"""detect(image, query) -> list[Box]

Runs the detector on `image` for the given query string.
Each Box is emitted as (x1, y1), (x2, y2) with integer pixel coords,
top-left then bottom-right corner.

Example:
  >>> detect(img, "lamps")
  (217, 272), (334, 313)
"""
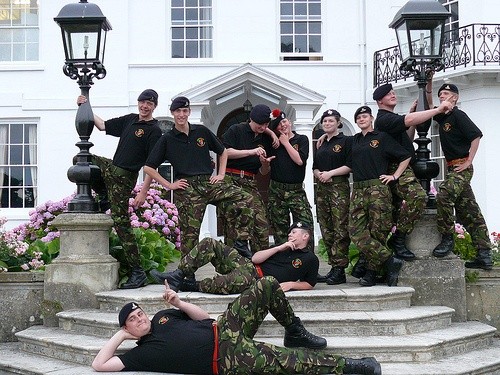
(244, 86), (253, 111)
(53, 0), (112, 213)
(388, 0), (451, 212)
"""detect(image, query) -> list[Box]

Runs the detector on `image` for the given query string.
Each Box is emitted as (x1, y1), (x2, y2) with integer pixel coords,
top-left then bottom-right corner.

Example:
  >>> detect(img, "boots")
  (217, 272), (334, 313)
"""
(387, 227), (416, 261)
(386, 254), (403, 286)
(351, 265), (364, 278)
(150, 266), (185, 292)
(344, 353), (381, 374)
(234, 238), (253, 258)
(432, 232), (454, 257)
(120, 264), (148, 287)
(317, 266), (346, 284)
(98, 187), (111, 212)
(464, 248), (493, 269)
(283, 317), (327, 349)
(359, 268), (377, 285)
(179, 278), (201, 291)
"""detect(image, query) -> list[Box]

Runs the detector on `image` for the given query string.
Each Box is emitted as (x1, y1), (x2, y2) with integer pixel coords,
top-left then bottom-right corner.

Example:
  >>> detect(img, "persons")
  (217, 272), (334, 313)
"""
(71, 88), (163, 289)
(353, 83), (452, 277)
(218, 104), (314, 252)
(312, 109), (353, 285)
(150, 222), (319, 297)
(316, 106), (412, 287)
(426, 79), (493, 271)
(144, 96), (255, 285)
(92, 275), (382, 375)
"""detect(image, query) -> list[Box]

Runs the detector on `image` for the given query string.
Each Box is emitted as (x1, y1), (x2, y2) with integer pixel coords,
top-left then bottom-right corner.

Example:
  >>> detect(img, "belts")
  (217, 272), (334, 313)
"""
(225, 172), (253, 179)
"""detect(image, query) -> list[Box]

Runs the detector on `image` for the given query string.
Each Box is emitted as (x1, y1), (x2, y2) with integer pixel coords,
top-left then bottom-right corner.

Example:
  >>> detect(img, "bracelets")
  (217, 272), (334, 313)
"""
(392, 175), (396, 180)
(426, 90), (432, 93)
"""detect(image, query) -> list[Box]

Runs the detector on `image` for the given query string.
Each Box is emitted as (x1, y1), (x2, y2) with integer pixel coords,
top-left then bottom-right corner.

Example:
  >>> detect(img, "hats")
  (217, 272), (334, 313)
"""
(373, 82), (391, 100)
(354, 105), (371, 122)
(170, 96), (190, 110)
(138, 88), (158, 101)
(269, 109), (286, 130)
(250, 103), (271, 124)
(119, 301), (139, 327)
(293, 220), (309, 227)
(437, 83), (458, 97)
(320, 109), (341, 123)
